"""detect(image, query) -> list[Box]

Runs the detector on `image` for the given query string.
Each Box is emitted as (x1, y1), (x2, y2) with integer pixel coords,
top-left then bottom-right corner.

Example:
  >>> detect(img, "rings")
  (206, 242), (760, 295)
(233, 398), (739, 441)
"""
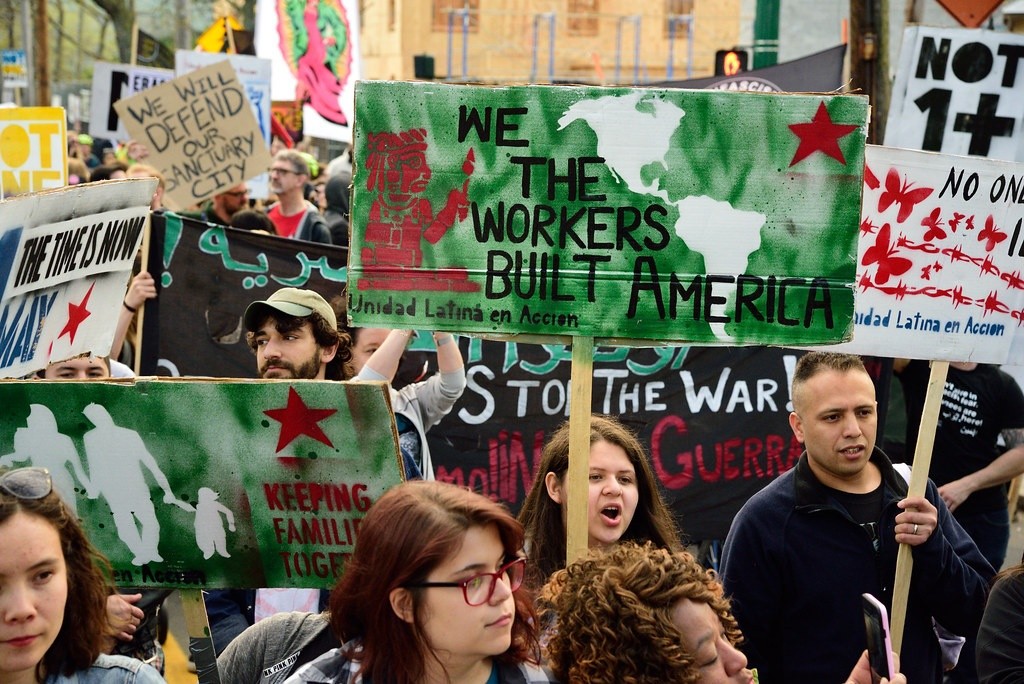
(913, 524), (918, 535)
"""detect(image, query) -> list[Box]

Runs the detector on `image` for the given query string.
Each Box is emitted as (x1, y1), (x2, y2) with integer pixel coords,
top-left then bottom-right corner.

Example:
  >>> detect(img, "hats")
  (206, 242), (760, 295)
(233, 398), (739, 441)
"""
(78, 134), (93, 145)
(244, 288), (337, 333)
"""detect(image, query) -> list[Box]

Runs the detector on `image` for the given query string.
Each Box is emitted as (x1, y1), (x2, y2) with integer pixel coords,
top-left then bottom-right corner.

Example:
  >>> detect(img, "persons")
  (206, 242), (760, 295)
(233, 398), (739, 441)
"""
(711, 349), (1002, 684)
(892, 358), (1024, 576)
(518, 414), (689, 635)
(976, 551), (1024, 684)
(1, 135), (547, 684)
(533, 539), (756, 684)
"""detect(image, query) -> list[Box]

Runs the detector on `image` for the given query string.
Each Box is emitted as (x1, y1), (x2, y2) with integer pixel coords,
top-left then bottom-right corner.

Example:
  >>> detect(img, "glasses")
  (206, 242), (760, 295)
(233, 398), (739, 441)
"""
(270, 168), (299, 177)
(0, 467), (52, 500)
(404, 557), (526, 606)
(226, 189), (247, 196)
